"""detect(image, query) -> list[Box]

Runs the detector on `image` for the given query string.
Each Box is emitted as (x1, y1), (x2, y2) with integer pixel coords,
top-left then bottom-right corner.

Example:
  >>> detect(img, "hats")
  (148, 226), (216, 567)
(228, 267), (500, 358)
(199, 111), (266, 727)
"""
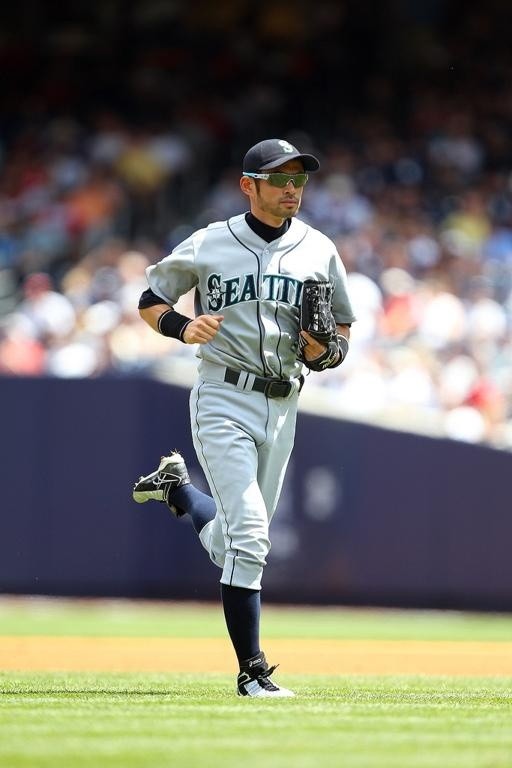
(244, 138), (321, 173)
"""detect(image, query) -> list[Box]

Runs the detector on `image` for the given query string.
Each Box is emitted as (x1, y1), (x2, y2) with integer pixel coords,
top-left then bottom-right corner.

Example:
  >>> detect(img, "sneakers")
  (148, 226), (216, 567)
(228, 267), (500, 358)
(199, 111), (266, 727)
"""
(236, 651), (293, 698)
(132, 454), (193, 519)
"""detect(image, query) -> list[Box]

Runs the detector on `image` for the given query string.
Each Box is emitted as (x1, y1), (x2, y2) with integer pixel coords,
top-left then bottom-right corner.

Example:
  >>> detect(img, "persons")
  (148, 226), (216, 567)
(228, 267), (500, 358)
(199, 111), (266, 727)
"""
(0, 1), (512, 452)
(131, 136), (356, 698)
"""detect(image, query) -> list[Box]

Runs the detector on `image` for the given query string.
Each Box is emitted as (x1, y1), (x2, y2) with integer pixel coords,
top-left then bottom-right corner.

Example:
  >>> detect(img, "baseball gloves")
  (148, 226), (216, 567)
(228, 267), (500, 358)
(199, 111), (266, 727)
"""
(298, 279), (338, 343)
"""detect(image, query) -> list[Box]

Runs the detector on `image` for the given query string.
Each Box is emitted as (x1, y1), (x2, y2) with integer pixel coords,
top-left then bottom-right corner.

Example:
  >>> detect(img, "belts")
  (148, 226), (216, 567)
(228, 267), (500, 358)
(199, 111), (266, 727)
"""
(224, 366), (304, 397)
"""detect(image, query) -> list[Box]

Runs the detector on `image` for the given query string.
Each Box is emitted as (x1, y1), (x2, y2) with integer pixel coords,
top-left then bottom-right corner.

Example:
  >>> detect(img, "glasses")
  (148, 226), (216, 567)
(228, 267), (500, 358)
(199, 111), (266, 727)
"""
(251, 173), (310, 188)
